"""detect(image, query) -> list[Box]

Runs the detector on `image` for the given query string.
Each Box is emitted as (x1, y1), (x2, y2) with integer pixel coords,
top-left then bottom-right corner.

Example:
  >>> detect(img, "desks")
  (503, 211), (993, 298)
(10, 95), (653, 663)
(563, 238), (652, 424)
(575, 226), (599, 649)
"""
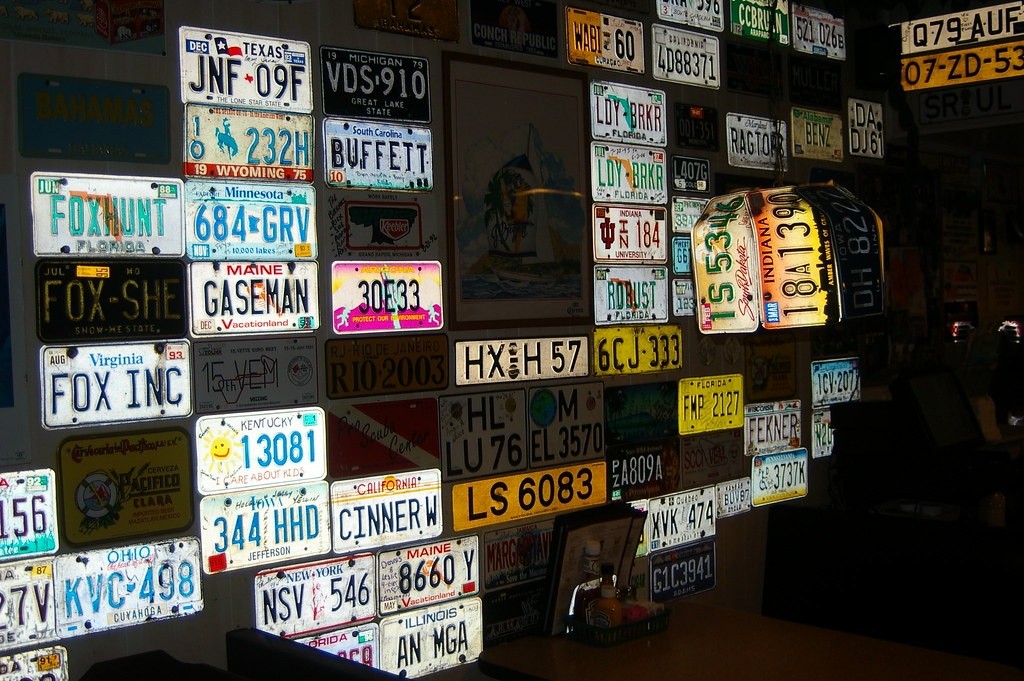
(760, 498), (1024, 672)
(478, 601), (1024, 681)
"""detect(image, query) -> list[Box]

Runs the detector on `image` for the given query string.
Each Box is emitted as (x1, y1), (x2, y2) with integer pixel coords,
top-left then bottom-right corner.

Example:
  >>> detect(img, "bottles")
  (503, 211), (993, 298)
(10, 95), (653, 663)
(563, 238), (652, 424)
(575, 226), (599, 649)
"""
(592, 585), (623, 642)
(573, 539), (603, 625)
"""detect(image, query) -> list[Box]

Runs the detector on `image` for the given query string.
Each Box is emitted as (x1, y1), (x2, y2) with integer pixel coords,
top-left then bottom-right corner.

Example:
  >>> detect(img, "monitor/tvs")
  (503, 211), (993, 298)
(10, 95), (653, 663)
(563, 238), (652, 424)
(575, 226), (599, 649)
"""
(892, 365), (986, 462)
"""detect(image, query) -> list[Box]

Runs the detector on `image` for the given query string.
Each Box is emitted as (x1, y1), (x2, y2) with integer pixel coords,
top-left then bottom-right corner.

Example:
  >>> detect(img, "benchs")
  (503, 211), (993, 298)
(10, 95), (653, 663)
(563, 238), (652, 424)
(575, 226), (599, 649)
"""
(225, 627), (413, 681)
